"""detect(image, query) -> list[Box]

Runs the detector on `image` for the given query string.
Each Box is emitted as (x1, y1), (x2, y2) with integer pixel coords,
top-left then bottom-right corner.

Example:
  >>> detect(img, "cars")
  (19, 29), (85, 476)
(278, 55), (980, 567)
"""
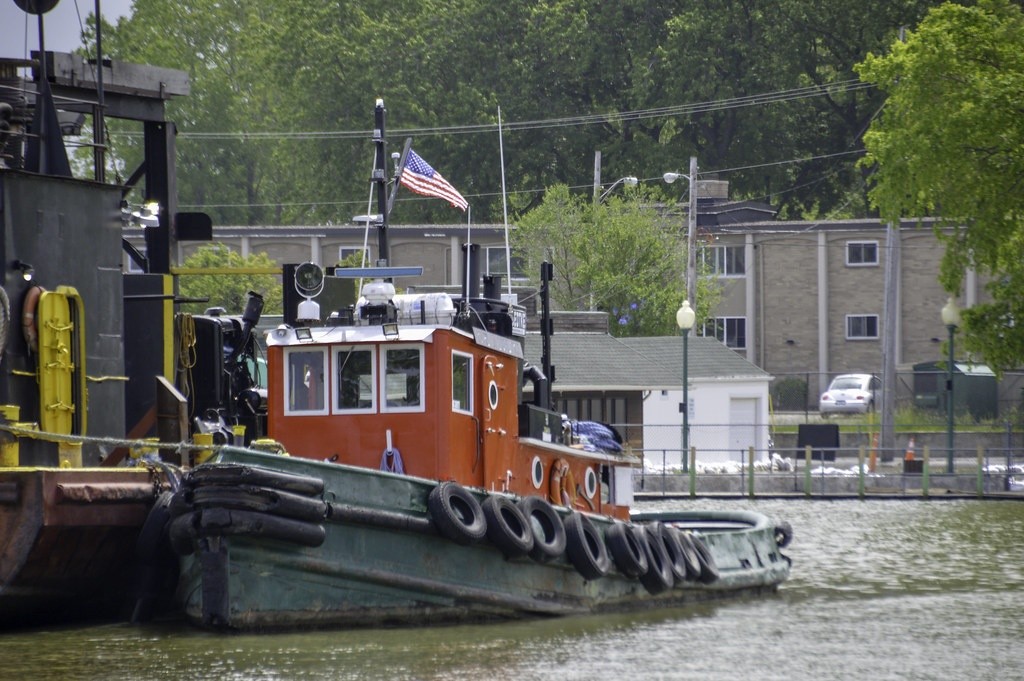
(818, 373), (886, 417)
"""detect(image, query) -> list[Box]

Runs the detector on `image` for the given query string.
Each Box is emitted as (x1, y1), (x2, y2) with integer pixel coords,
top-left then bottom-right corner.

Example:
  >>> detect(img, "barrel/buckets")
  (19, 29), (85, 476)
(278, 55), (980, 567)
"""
(194, 434), (213, 463)
(0, 404), (19, 468)
(131, 438), (160, 467)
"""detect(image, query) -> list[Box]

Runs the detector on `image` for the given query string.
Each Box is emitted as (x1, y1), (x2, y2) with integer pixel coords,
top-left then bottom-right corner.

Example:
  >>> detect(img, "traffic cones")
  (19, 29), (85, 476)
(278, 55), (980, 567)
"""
(904, 437), (919, 461)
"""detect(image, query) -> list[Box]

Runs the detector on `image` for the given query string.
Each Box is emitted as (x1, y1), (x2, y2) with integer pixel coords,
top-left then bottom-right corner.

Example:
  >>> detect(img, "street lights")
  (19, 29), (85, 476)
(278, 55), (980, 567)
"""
(589, 149), (637, 311)
(662, 156), (700, 339)
(939, 297), (961, 476)
(675, 299), (697, 470)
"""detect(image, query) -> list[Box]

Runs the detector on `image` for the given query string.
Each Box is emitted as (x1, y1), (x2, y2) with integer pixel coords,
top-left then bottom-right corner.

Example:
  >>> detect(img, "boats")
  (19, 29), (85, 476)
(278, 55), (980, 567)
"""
(134, 100), (797, 631)
(1, 1), (272, 615)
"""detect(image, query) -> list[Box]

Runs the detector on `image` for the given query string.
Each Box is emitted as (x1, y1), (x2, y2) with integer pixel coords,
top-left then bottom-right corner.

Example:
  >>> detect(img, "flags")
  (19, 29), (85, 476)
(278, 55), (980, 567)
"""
(398, 147), (469, 213)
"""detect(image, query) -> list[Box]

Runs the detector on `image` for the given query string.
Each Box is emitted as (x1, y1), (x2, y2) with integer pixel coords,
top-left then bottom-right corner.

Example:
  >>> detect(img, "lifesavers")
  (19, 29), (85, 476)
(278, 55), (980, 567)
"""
(427, 477), (490, 543)
(650, 520), (687, 585)
(484, 495), (536, 563)
(21, 283), (49, 347)
(634, 524), (675, 595)
(669, 525), (701, 585)
(566, 511), (610, 581)
(516, 494), (567, 567)
(606, 522), (649, 578)
(687, 532), (719, 585)
